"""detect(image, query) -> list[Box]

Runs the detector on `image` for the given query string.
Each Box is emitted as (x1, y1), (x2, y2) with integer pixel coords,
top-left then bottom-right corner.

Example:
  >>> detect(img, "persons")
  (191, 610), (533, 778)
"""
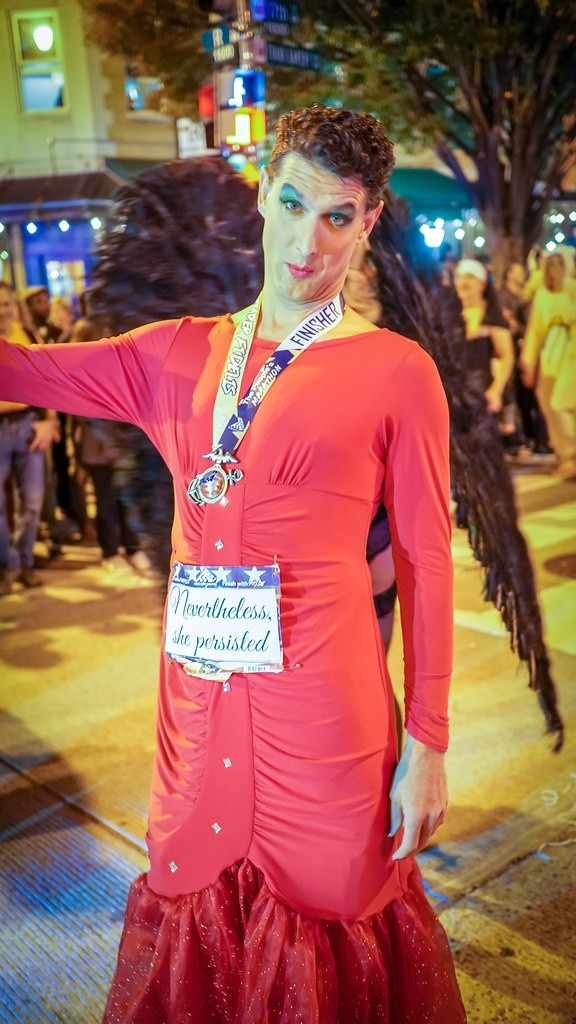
(0, 103), (461, 1009)
(0, 219), (576, 594)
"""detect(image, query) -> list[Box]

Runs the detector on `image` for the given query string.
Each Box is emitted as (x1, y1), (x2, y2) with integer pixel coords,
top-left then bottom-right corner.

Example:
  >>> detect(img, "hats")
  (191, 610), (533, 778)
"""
(455, 259), (487, 281)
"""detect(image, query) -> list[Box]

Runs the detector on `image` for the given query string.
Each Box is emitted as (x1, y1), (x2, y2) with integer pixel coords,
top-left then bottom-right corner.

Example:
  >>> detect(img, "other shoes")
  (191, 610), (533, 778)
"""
(129, 550), (152, 569)
(0, 565), (21, 596)
(532, 444), (558, 461)
(100, 554), (133, 575)
(18, 567), (43, 587)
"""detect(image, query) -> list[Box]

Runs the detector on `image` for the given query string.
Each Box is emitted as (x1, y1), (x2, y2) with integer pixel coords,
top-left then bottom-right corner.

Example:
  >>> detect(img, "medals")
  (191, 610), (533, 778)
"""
(184, 448), (246, 510)
